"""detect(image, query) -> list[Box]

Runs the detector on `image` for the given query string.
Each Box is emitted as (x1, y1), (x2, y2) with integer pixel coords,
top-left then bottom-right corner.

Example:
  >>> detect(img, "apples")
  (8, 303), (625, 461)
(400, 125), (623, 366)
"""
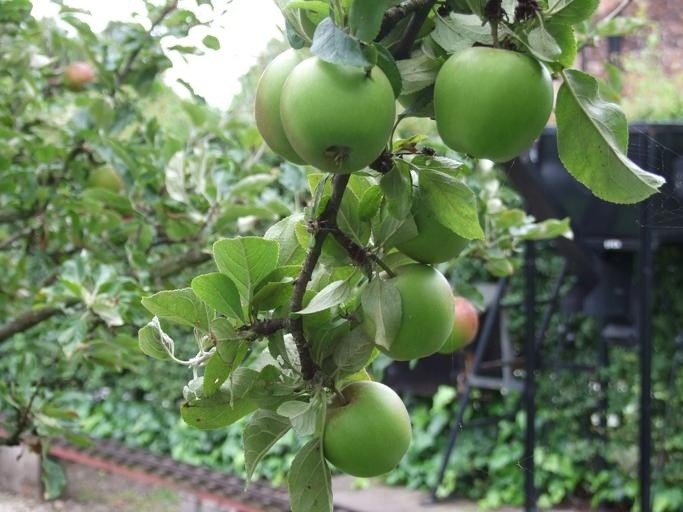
(66, 61), (92, 88)
(276, 50), (395, 176)
(440, 293), (478, 358)
(318, 381), (412, 480)
(254, 47), (322, 167)
(430, 45), (555, 167)
(362, 261), (459, 361)
(88, 163), (123, 193)
